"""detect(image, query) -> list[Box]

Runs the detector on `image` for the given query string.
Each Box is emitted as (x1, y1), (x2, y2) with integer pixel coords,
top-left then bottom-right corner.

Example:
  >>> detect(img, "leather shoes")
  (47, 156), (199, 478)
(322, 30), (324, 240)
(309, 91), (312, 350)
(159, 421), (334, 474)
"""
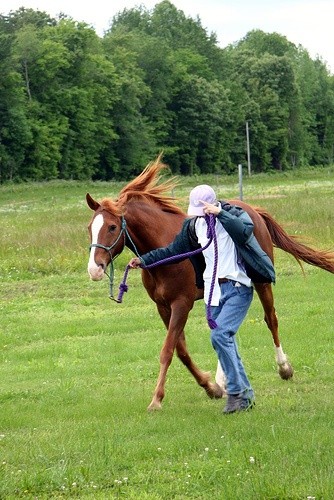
(237, 396), (256, 412)
(223, 393), (239, 415)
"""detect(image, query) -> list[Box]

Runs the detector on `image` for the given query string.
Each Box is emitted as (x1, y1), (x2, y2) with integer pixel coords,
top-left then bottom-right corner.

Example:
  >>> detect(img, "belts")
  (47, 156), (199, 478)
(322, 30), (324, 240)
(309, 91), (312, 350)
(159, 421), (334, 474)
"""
(218, 278), (231, 284)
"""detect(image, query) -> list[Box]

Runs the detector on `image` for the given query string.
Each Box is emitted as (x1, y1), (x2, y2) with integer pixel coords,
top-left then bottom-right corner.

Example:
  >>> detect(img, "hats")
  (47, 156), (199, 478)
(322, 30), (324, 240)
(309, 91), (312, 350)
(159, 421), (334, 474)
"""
(187, 184), (216, 218)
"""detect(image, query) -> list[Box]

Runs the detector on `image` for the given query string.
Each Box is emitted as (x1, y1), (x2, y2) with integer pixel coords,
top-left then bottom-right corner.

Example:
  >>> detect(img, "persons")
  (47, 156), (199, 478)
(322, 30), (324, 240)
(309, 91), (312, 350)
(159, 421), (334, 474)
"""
(128, 185), (276, 413)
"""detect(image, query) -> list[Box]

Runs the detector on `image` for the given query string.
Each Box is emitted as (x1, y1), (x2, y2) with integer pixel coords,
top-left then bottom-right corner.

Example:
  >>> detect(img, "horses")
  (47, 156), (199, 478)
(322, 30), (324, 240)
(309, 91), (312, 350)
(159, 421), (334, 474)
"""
(86, 149), (333, 413)
